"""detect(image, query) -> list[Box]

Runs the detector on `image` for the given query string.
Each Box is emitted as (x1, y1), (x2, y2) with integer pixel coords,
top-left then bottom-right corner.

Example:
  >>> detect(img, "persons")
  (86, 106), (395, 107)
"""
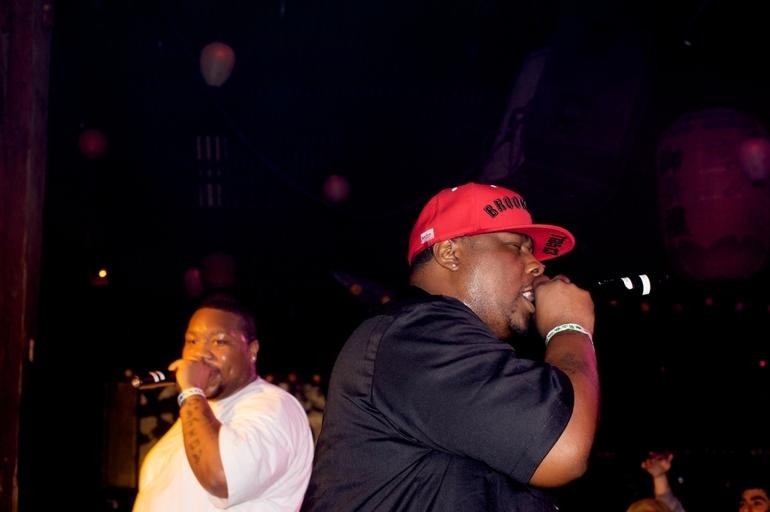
(624, 497), (672, 512)
(132, 293), (317, 512)
(641, 450), (770, 512)
(300, 181), (602, 512)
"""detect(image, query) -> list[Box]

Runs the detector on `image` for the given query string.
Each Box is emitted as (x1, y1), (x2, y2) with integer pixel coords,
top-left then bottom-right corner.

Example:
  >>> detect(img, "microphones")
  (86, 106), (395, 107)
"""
(572, 271), (658, 309)
(131, 370), (178, 391)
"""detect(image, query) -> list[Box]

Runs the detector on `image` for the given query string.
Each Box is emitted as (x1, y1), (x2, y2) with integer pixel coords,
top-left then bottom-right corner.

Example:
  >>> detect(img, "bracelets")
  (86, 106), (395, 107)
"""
(177, 387), (206, 406)
(545, 323), (595, 350)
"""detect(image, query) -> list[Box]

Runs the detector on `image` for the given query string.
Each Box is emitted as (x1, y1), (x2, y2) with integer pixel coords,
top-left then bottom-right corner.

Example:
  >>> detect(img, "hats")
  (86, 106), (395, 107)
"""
(406, 181), (577, 267)
